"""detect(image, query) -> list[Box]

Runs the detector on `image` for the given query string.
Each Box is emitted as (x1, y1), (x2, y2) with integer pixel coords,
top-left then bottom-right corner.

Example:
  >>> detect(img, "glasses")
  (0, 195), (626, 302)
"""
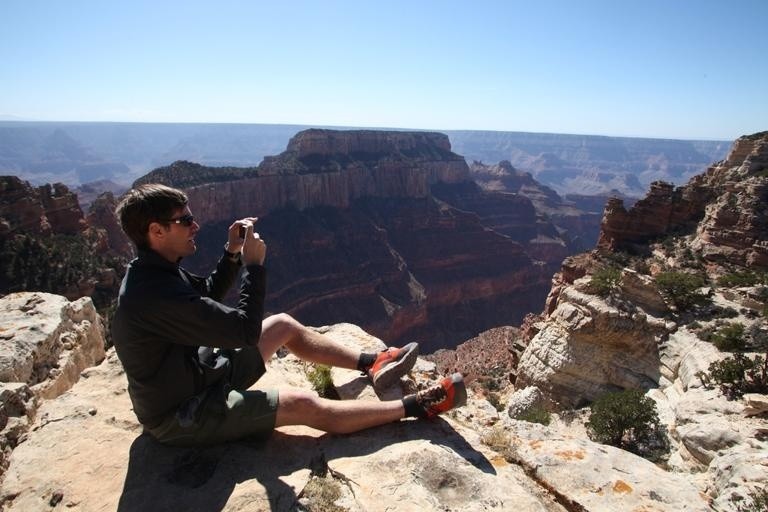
(163, 214), (194, 227)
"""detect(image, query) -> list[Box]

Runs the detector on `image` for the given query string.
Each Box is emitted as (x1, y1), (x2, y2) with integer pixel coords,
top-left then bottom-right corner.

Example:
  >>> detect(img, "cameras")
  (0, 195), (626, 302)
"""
(238, 224), (248, 239)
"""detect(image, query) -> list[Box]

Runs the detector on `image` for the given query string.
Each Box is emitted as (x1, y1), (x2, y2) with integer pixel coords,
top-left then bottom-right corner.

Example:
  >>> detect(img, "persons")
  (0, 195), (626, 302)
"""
(112, 183), (467, 447)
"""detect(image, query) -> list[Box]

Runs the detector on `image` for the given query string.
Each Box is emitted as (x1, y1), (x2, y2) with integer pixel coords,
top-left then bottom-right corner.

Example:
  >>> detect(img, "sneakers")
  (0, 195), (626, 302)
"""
(416, 372), (467, 418)
(367, 341), (420, 391)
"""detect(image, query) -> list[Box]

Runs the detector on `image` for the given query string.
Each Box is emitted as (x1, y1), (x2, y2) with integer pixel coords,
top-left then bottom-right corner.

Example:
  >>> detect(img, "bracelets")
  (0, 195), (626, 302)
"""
(222, 241), (241, 260)
